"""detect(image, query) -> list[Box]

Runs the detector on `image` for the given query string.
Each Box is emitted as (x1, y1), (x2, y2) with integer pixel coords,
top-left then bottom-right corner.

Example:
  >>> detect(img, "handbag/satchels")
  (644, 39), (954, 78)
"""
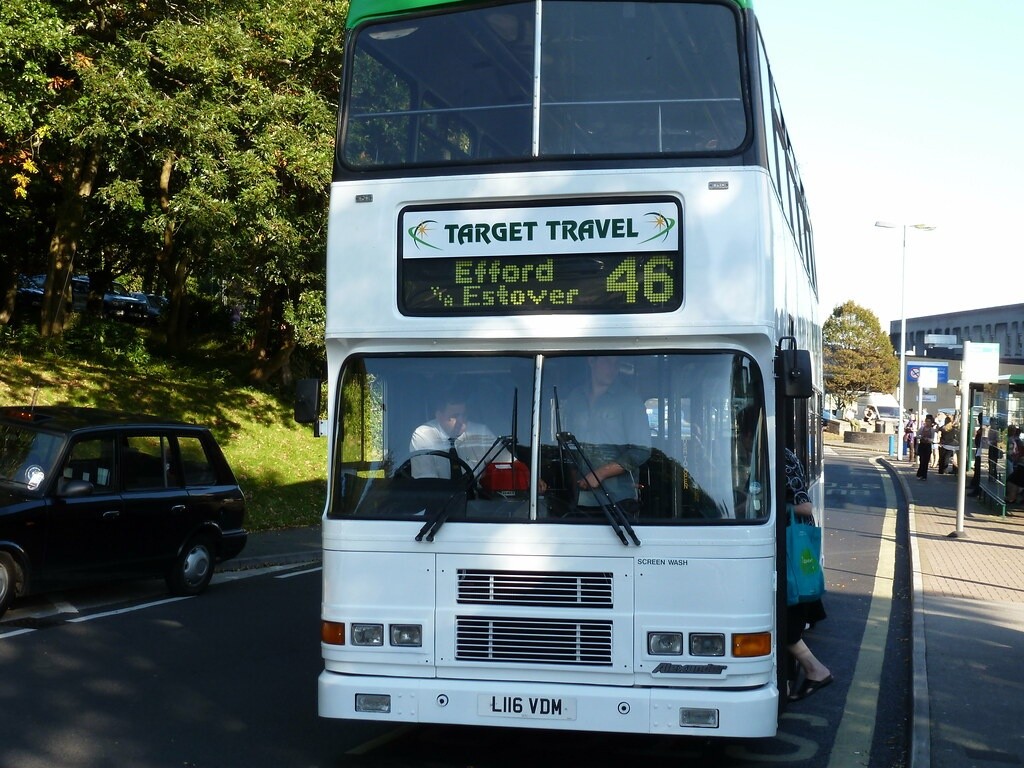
(915, 448), (918, 455)
(785, 504), (825, 606)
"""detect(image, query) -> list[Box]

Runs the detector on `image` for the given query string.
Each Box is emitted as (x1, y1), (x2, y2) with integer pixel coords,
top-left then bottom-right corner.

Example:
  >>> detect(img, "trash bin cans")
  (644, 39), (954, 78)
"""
(874, 420), (886, 433)
(938, 444), (955, 474)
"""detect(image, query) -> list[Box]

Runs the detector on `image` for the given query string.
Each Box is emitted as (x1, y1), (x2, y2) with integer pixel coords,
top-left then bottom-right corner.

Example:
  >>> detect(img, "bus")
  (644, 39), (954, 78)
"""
(294, 0), (825, 736)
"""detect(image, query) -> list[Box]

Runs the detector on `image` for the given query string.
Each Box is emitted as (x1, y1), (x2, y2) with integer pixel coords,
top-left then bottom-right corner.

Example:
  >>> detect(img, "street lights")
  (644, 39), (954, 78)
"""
(875, 220), (936, 461)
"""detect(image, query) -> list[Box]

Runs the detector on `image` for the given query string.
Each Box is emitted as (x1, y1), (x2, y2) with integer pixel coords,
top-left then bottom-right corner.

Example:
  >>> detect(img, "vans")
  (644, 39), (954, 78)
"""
(836, 391), (909, 434)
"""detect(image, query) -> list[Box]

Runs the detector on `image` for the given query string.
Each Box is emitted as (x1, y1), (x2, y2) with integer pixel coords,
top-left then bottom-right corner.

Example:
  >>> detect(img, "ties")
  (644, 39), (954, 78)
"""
(449, 437), (462, 480)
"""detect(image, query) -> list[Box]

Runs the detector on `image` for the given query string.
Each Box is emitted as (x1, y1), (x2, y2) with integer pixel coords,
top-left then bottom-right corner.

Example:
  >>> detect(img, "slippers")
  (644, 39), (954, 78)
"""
(787, 674), (835, 702)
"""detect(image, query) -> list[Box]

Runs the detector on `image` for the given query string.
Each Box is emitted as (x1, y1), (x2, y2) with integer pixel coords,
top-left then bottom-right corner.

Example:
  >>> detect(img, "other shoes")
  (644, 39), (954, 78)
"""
(917, 476), (920, 480)
(921, 477), (926, 481)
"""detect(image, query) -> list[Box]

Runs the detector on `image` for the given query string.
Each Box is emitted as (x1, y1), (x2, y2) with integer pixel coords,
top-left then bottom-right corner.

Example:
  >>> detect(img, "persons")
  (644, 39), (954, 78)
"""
(968, 411), (1024, 505)
(410, 377), (546, 517)
(565, 356), (651, 517)
(989, 417), (999, 481)
(934, 416), (958, 474)
(739, 406), (833, 701)
(846, 408), (854, 419)
(904, 408), (916, 461)
(551, 88), (624, 154)
(864, 408), (875, 425)
(915, 414), (934, 481)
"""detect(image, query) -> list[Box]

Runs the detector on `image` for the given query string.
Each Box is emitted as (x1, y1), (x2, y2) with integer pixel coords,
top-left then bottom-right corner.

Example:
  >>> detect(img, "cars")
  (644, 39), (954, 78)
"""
(18, 269), (149, 325)
(0, 405), (249, 617)
(124, 292), (167, 321)
